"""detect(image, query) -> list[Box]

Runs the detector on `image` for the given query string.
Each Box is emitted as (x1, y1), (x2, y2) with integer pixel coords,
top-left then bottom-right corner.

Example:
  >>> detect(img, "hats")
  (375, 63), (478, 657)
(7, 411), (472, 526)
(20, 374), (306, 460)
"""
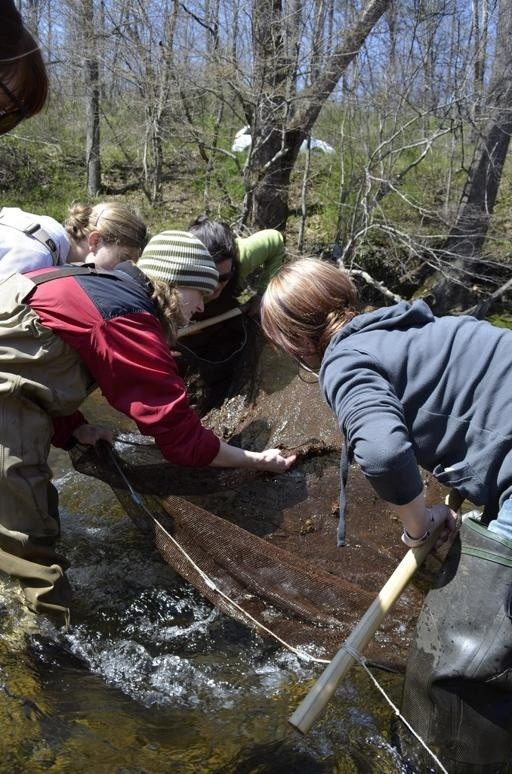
(136, 229), (219, 298)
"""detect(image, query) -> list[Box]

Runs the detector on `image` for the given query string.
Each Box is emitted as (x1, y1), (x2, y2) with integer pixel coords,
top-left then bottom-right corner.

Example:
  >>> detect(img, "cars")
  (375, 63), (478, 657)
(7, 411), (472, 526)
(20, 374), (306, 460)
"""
(232, 124), (335, 156)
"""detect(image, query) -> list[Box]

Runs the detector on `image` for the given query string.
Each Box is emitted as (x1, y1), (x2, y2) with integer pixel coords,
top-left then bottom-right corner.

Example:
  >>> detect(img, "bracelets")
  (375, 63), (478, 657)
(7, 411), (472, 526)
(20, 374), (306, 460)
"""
(403, 505), (435, 544)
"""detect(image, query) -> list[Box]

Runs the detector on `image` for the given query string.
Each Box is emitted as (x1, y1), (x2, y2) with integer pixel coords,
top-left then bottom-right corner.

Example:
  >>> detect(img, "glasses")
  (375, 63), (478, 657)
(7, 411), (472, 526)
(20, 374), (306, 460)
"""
(219, 265), (234, 282)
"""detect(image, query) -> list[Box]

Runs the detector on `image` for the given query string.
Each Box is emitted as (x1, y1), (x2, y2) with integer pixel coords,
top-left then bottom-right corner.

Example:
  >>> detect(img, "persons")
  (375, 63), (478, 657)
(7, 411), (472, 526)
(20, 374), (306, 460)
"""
(0, 0), (50, 135)
(1, 198), (148, 280)
(182, 212), (286, 395)
(256, 255), (512, 774)
(1, 228), (298, 628)
